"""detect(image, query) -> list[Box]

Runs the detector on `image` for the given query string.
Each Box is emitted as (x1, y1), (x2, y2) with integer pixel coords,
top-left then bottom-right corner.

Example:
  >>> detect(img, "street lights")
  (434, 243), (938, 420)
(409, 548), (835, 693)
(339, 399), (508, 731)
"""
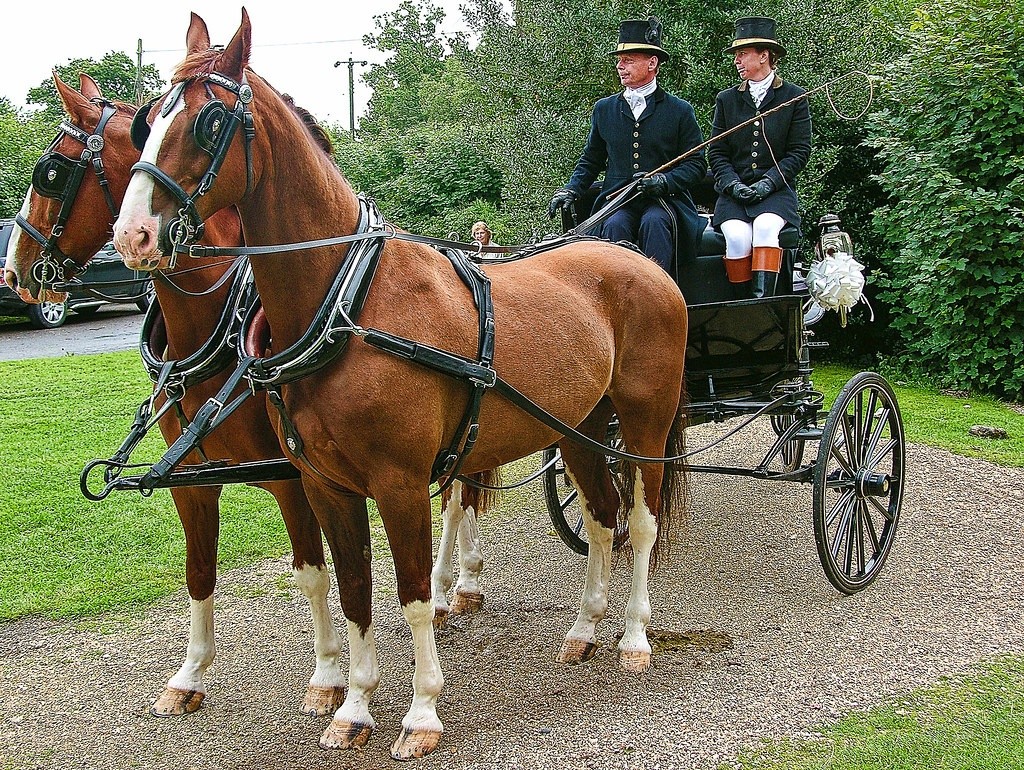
(335, 57), (368, 142)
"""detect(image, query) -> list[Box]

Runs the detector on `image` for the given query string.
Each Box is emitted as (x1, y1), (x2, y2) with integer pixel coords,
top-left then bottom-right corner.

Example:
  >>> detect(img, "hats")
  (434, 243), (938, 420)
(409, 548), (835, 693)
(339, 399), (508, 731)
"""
(607, 17), (670, 62)
(722, 16), (787, 57)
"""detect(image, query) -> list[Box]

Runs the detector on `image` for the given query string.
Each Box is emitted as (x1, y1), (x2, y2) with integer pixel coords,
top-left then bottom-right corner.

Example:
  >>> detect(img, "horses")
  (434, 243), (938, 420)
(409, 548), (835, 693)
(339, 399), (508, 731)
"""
(3, 7), (690, 760)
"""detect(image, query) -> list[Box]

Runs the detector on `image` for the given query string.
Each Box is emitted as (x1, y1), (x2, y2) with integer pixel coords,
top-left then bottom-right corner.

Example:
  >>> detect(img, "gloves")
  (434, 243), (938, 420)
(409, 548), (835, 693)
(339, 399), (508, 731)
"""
(548, 190), (575, 219)
(731, 179), (772, 205)
(633, 171), (667, 198)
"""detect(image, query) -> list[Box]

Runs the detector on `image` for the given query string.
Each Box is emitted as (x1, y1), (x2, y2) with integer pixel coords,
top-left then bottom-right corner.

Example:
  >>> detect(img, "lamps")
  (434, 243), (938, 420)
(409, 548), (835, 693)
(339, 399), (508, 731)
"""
(815, 213), (852, 263)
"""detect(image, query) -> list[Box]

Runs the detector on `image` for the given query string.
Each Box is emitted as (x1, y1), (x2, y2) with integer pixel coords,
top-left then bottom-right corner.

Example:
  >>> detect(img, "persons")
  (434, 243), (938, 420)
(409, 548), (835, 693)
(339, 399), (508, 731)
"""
(708, 18), (813, 300)
(549, 19), (709, 273)
(466, 222), (503, 265)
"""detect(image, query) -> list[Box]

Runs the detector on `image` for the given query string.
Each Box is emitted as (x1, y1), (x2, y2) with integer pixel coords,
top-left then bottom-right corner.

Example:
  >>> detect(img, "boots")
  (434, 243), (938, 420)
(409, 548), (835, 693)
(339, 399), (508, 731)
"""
(722, 246), (783, 301)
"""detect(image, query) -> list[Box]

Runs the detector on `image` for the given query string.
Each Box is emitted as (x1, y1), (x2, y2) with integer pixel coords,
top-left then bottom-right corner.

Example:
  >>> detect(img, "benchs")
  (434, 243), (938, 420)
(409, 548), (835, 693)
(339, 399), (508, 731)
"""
(560, 169), (808, 394)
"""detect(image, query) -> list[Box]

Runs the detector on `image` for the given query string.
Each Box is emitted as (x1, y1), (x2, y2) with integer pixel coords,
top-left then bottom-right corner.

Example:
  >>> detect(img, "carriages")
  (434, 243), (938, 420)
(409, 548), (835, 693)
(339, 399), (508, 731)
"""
(4, 5), (906, 761)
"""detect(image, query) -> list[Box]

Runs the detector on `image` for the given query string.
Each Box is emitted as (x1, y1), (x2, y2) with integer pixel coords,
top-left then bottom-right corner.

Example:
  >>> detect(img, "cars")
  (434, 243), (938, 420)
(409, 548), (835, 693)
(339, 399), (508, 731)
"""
(0, 218), (157, 329)
